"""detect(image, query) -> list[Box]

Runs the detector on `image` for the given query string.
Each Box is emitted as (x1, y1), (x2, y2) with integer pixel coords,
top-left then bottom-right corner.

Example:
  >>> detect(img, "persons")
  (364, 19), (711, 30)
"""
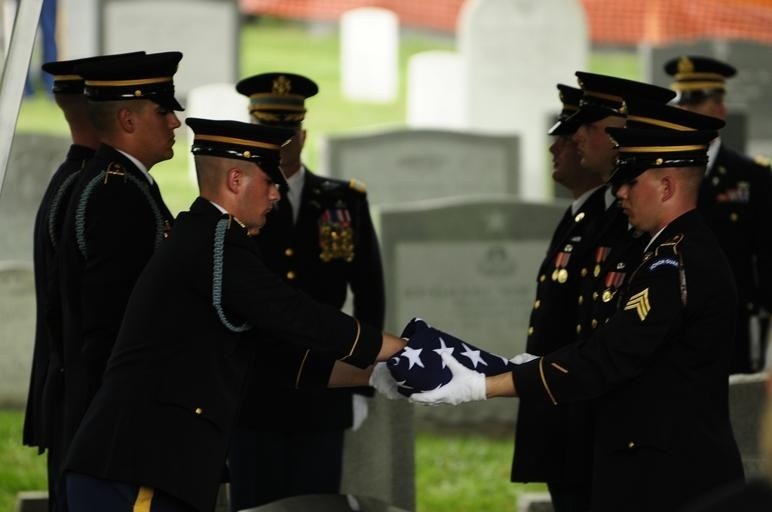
(509, 56), (772, 512)
(64, 117), (407, 511)
(410, 125), (748, 511)
(24, 51), (185, 511)
(236, 72), (387, 500)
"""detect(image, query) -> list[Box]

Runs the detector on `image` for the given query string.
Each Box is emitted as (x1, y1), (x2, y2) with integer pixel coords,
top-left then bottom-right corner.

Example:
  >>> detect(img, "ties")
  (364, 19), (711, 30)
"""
(277, 184), (293, 226)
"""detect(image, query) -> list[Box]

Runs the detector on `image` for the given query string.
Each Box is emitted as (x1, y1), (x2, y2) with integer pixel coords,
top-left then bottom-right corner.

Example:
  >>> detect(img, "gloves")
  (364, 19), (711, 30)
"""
(367, 359), (409, 401)
(406, 350), (488, 408)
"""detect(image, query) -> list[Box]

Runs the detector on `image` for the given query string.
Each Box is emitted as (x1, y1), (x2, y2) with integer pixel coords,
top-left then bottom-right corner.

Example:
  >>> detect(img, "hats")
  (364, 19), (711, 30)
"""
(41, 52), (146, 95)
(547, 83), (584, 136)
(565, 71), (677, 125)
(663, 56), (739, 104)
(605, 126), (711, 185)
(235, 72), (320, 125)
(83, 51), (186, 112)
(622, 100), (726, 136)
(184, 117), (301, 166)
